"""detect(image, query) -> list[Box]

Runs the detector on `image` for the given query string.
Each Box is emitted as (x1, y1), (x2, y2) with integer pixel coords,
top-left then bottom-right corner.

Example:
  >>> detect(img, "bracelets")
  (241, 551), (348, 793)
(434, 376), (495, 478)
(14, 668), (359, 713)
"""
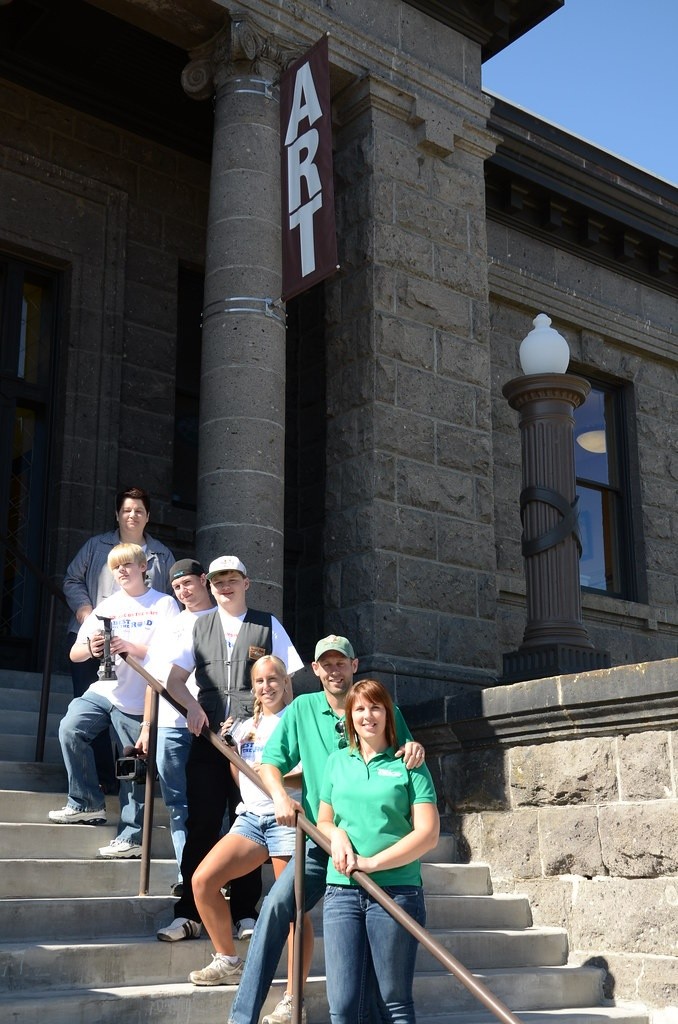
(140, 722), (150, 727)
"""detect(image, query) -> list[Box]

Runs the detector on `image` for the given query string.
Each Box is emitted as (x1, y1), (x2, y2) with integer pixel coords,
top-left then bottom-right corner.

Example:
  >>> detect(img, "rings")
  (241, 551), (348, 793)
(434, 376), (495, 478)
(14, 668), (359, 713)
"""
(220, 722), (224, 726)
(417, 754), (421, 757)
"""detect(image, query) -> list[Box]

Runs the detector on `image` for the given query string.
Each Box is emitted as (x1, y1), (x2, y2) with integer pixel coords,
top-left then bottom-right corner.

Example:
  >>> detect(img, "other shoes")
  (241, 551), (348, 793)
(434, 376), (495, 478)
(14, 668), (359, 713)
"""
(170, 881), (184, 896)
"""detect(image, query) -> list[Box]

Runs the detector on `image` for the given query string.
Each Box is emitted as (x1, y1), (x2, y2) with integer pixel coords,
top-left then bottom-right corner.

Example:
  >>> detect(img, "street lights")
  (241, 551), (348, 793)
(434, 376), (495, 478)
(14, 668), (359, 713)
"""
(502, 313), (610, 684)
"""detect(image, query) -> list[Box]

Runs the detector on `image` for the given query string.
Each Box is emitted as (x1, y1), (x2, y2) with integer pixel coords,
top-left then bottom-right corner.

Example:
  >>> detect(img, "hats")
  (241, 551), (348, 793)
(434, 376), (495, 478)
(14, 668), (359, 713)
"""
(205, 556), (246, 582)
(315, 634), (354, 662)
(169, 558), (204, 583)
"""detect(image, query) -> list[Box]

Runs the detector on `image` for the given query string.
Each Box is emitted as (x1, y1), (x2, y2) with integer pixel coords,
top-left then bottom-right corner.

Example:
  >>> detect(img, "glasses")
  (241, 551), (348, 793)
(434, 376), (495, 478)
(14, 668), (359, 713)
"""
(335, 721), (347, 750)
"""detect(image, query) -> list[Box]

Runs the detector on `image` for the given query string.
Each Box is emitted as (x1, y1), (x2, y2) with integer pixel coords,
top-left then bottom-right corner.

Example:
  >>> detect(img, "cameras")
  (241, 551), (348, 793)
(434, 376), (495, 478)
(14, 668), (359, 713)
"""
(223, 717), (247, 747)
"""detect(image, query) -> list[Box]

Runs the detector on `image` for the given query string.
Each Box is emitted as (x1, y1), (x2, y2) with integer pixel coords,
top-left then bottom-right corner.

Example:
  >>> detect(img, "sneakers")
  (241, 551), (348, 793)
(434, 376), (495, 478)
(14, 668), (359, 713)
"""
(48, 805), (107, 824)
(189, 953), (245, 985)
(95, 839), (142, 858)
(262, 992), (308, 1024)
(156, 918), (202, 942)
(235, 918), (256, 940)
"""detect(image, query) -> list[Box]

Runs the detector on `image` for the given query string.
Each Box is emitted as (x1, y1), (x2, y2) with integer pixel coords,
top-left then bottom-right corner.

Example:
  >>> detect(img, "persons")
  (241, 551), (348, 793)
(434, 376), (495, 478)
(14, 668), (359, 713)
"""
(49, 543), (181, 860)
(190, 655), (313, 1024)
(227, 635), (425, 1024)
(157, 556), (304, 943)
(135, 559), (231, 899)
(318, 679), (441, 1024)
(62, 488), (176, 794)
